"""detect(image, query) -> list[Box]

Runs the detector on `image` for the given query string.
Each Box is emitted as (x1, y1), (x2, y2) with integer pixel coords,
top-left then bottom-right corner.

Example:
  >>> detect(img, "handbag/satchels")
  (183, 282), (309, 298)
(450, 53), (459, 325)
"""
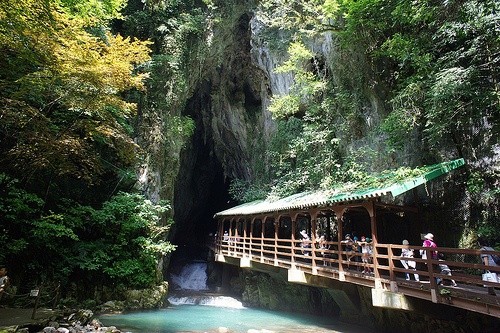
(481, 270), (498, 288)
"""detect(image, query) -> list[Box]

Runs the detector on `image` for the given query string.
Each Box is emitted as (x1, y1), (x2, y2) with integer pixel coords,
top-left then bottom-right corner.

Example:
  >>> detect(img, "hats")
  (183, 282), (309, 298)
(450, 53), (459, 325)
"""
(424, 233), (434, 241)
(300, 230), (306, 235)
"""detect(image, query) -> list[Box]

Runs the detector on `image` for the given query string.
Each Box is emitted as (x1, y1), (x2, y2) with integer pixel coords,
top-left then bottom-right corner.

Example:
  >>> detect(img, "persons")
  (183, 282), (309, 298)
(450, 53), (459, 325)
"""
(230, 235), (234, 251)
(299, 230), (310, 264)
(477, 237), (500, 296)
(351, 232), (364, 271)
(236, 235), (240, 252)
(357, 236), (368, 273)
(341, 234), (356, 269)
(224, 231), (229, 250)
(438, 253), (457, 286)
(399, 240), (420, 282)
(419, 233), (442, 285)
(319, 236), (332, 267)
(366, 236), (375, 273)
